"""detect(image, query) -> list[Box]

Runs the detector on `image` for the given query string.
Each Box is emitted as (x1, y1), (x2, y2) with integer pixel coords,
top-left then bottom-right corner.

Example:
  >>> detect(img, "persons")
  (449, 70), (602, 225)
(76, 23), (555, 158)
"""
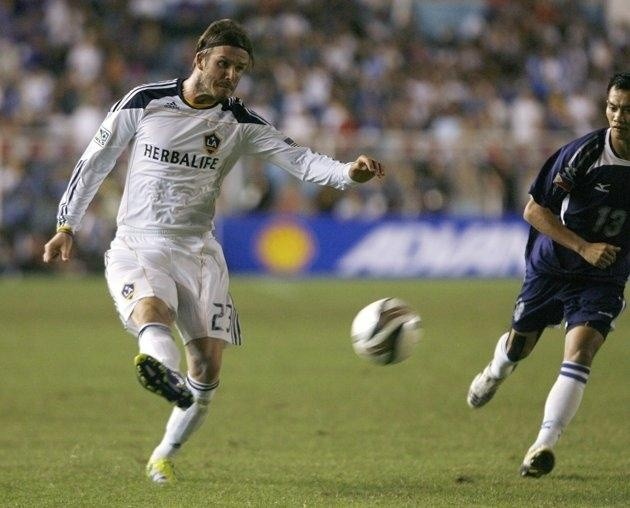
(1, 0), (630, 281)
(467, 70), (630, 479)
(43, 16), (385, 482)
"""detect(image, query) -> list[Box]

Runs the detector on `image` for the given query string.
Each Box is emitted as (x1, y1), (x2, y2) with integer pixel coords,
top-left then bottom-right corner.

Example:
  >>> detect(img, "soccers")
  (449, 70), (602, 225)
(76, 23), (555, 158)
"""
(349, 296), (422, 367)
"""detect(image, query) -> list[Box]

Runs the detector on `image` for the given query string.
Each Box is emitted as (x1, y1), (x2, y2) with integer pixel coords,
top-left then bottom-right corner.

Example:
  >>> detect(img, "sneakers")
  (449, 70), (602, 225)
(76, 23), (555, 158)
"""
(464, 356), (520, 410)
(145, 453), (176, 485)
(517, 444), (556, 480)
(131, 353), (197, 413)
